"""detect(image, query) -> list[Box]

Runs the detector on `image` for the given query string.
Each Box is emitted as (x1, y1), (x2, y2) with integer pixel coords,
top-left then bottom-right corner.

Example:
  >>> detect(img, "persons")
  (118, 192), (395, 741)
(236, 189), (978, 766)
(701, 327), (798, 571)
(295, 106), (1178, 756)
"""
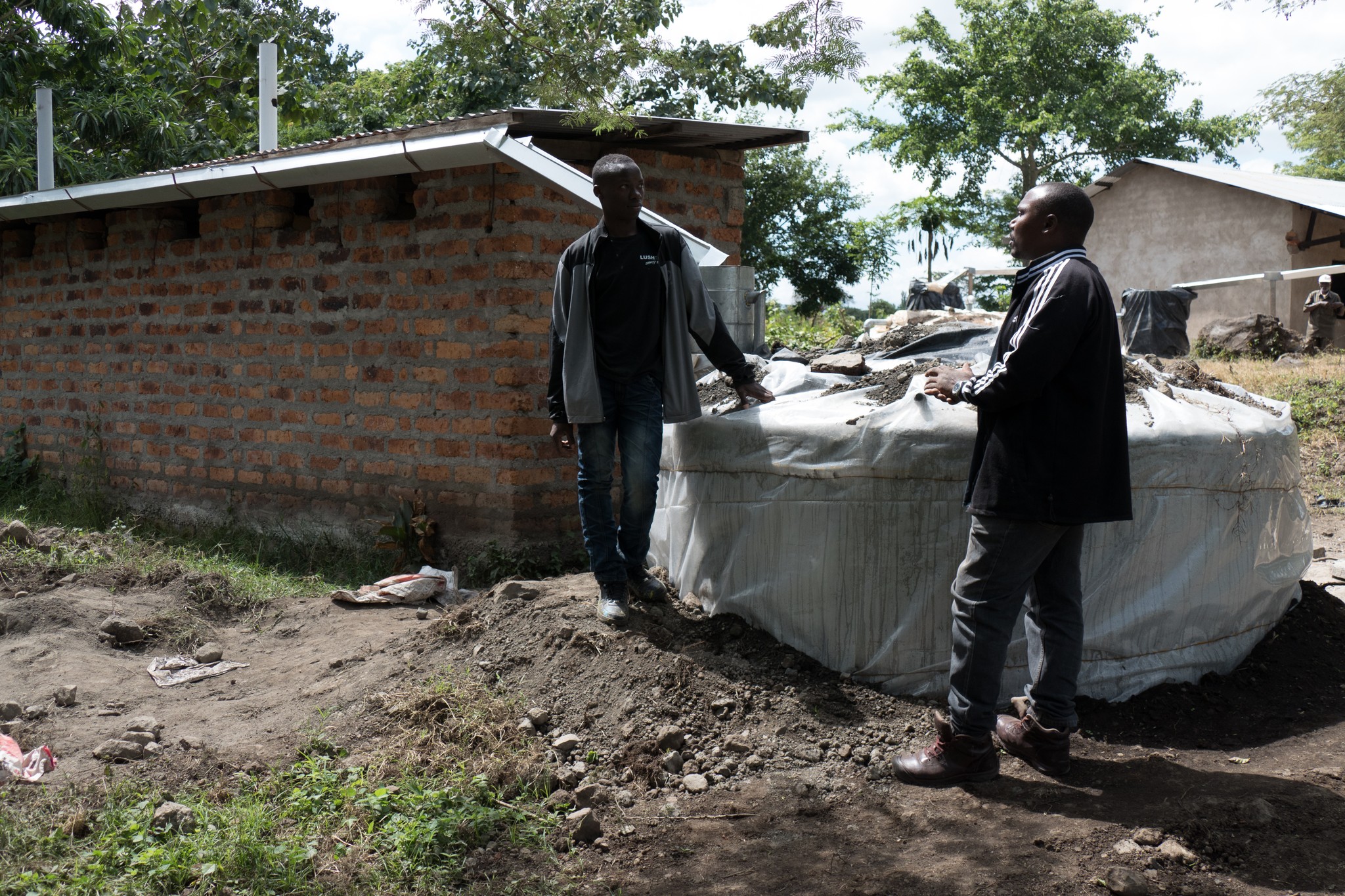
(547, 153), (775, 625)
(894, 181), (1131, 787)
(1302, 274), (1345, 354)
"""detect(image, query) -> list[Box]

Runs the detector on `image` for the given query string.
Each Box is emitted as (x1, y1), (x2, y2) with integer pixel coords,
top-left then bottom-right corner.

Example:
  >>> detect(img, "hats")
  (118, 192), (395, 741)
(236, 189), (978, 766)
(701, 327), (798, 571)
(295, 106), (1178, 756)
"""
(1318, 274), (1331, 282)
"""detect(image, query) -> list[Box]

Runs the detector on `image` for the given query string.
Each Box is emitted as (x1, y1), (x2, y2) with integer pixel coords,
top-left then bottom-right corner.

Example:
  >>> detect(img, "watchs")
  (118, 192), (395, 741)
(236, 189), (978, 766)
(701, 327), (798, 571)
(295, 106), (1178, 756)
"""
(952, 380), (970, 404)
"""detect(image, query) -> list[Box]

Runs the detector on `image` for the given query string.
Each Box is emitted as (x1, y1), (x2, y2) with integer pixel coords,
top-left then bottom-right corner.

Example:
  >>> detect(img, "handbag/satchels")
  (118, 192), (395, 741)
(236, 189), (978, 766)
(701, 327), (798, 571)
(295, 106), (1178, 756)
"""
(1120, 286), (1198, 359)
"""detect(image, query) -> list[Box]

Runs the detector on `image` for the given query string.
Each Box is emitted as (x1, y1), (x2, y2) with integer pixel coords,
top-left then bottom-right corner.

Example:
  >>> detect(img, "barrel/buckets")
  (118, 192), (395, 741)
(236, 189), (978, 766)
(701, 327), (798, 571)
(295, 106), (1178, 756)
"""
(688, 266), (755, 354)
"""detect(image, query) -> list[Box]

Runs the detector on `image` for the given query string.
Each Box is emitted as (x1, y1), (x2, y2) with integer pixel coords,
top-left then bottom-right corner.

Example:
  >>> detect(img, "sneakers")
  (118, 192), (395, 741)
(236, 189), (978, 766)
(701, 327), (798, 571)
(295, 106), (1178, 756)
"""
(627, 568), (667, 602)
(995, 696), (1072, 776)
(891, 710), (1000, 786)
(597, 581), (630, 625)
